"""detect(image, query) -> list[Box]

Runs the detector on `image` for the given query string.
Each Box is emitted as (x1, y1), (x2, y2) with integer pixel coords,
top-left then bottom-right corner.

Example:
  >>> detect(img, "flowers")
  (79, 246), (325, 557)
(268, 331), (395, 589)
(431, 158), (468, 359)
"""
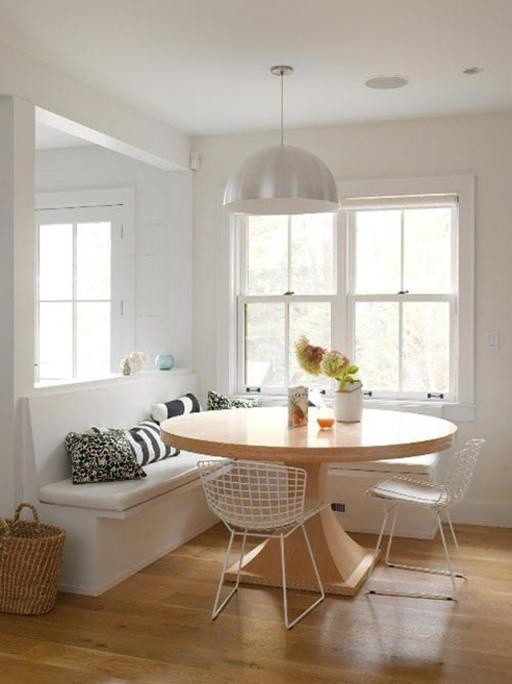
(295, 335), (359, 384)
(120, 352), (144, 373)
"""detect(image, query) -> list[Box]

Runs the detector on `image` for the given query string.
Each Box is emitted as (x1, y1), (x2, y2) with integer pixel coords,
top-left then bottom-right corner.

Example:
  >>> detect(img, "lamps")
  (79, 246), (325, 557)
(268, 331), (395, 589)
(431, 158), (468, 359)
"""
(222, 65), (339, 216)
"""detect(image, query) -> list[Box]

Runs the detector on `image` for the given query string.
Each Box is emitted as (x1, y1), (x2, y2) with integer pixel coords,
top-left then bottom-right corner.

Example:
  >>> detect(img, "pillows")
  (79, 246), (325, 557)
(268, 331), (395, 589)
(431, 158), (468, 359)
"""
(90, 417), (180, 467)
(65, 430), (146, 484)
(151, 394), (202, 425)
(207, 390), (260, 410)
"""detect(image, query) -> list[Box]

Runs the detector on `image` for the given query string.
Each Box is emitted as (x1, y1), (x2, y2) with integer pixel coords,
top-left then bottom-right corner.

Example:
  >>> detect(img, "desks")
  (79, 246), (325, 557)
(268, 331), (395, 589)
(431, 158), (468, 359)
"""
(160, 406), (458, 598)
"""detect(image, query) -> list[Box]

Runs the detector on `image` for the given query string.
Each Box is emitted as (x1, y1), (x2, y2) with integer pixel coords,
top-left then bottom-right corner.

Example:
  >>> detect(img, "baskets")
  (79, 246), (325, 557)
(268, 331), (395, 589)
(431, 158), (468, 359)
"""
(2, 503), (66, 616)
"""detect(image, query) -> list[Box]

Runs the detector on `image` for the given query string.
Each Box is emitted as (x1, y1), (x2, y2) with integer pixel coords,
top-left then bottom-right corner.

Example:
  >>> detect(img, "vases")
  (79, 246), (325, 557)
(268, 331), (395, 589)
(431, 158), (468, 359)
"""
(336, 380), (363, 424)
(123, 363), (131, 375)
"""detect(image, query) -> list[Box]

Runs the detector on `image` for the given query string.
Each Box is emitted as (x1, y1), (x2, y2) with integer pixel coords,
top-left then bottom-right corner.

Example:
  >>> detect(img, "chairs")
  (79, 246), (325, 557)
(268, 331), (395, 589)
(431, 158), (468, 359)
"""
(363, 438), (486, 603)
(198, 459), (330, 630)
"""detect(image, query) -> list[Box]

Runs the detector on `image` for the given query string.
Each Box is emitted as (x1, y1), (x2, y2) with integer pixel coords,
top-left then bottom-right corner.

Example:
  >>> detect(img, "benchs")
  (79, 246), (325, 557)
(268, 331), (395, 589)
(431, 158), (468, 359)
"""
(234, 401), (443, 540)
(20, 374), (235, 598)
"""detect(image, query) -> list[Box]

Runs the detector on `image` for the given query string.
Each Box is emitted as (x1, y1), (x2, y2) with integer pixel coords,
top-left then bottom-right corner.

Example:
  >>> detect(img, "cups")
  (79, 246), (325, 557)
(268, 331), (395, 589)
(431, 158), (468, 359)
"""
(317, 399), (335, 429)
